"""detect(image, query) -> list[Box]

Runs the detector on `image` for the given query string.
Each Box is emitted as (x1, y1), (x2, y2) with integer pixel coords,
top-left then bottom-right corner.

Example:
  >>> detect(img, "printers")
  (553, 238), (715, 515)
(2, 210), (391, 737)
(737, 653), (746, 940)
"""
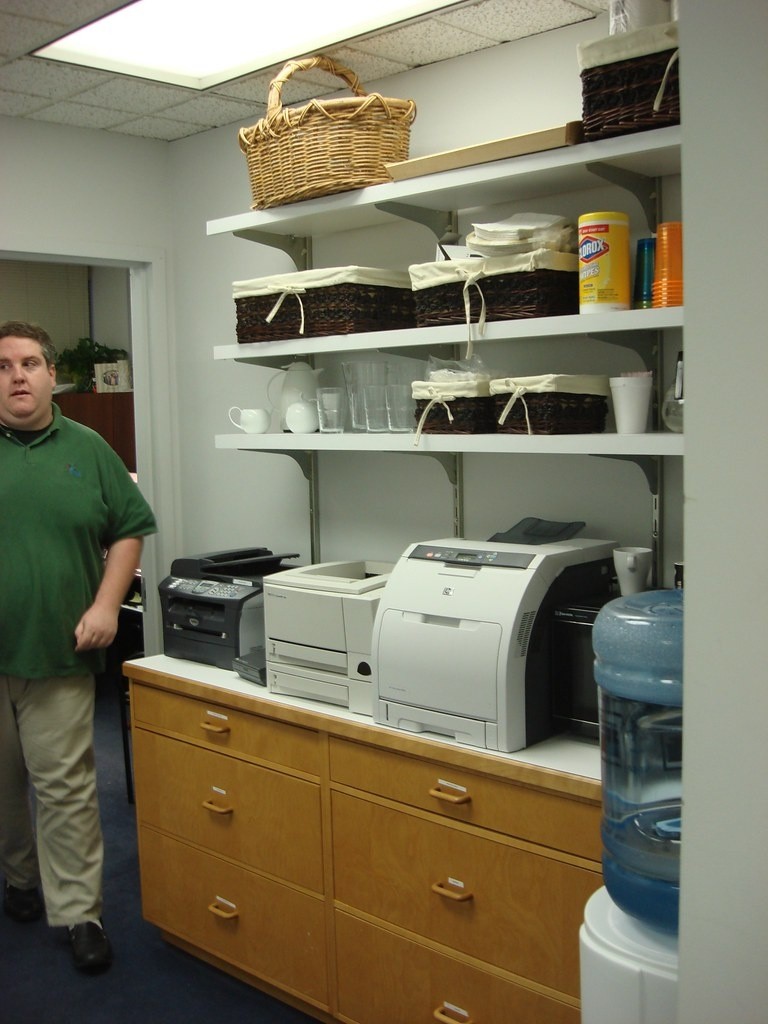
(262, 560), (396, 716)
(371, 517), (621, 754)
(158, 547), (303, 671)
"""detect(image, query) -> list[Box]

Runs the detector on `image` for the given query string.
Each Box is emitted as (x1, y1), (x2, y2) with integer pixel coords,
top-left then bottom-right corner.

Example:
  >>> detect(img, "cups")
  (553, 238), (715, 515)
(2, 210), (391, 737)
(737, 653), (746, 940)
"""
(317, 387), (345, 433)
(613, 547), (652, 597)
(228, 406), (270, 434)
(340, 361), (425, 433)
(609, 377), (652, 433)
(633, 221), (684, 310)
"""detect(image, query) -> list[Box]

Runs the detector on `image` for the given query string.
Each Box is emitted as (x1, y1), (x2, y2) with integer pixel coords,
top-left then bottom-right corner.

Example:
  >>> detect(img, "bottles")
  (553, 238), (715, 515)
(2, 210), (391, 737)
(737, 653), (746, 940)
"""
(674, 562), (683, 589)
(593, 588), (683, 939)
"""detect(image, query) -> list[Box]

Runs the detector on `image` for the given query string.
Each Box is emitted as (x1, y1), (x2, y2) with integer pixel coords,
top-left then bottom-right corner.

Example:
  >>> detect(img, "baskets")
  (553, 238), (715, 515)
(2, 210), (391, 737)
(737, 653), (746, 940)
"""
(409, 378), (497, 434)
(407, 249), (578, 328)
(579, 21), (680, 141)
(489, 374), (608, 435)
(234, 264), (415, 344)
(234, 55), (415, 211)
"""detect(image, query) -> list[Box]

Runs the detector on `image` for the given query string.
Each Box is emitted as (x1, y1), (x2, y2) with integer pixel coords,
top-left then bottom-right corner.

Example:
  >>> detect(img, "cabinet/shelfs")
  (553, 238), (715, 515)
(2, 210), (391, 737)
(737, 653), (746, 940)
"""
(206, 125), (684, 456)
(128, 675), (606, 1024)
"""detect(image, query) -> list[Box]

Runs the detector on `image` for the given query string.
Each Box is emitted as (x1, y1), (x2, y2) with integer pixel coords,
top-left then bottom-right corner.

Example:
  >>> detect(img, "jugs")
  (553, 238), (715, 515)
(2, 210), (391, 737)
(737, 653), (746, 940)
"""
(661, 350), (685, 431)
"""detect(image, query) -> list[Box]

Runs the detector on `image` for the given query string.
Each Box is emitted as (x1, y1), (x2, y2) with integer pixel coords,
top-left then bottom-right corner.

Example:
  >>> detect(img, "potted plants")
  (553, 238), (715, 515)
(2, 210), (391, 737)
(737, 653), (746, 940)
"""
(56, 338), (128, 391)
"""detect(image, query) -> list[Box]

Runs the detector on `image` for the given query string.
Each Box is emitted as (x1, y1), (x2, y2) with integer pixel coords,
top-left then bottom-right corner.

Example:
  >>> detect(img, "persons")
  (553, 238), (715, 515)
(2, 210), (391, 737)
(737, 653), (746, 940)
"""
(0, 320), (159, 976)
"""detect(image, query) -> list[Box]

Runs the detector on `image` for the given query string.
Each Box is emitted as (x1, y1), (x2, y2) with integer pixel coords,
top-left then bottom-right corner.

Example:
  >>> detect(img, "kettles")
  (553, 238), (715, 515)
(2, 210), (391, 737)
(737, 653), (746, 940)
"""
(266, 362), (325, 433)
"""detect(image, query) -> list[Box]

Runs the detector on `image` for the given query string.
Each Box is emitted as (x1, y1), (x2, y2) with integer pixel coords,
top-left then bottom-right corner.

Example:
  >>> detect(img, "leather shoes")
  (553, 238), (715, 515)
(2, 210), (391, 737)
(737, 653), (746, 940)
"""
(69, 917), (112, 974)
(4, 881), (44, 922)
